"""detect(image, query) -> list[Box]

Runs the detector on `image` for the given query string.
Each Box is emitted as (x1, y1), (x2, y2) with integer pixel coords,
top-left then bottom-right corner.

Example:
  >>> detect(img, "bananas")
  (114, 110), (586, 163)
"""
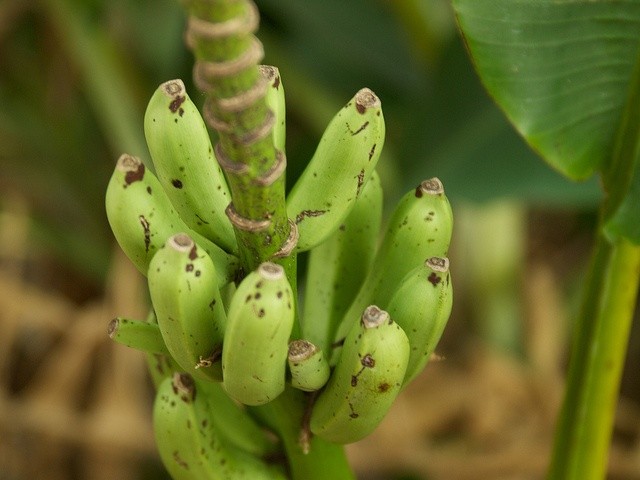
(103, 77), (456, 480)
